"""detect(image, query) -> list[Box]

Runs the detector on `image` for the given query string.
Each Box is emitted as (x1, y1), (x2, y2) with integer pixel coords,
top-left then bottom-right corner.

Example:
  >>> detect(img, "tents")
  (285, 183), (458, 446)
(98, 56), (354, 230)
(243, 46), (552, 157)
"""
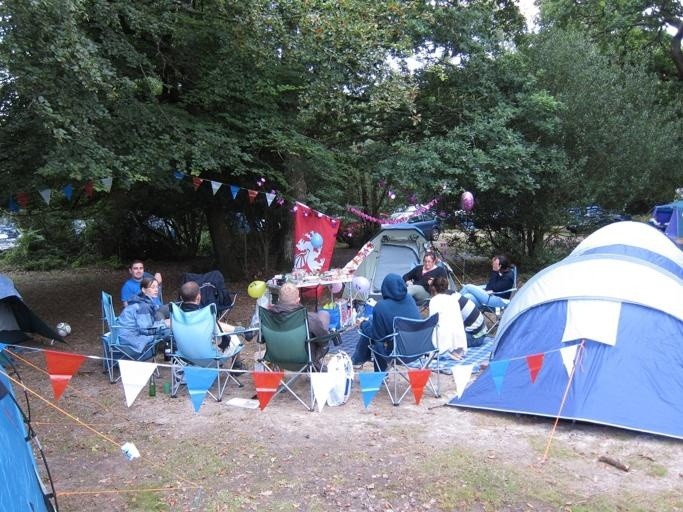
(0, 270), (67, 512)
(343, 221), (447, 300)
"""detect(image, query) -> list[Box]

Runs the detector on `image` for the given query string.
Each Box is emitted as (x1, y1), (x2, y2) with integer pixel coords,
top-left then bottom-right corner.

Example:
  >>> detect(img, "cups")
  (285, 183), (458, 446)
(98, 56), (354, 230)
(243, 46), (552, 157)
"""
(162, 383), (170, 394)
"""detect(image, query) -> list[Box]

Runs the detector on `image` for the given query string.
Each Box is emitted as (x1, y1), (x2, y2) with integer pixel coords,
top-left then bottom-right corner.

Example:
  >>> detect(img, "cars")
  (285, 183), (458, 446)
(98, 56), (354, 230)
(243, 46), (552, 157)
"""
(567, 205), (631, 234)
(385, 211), (441, 241)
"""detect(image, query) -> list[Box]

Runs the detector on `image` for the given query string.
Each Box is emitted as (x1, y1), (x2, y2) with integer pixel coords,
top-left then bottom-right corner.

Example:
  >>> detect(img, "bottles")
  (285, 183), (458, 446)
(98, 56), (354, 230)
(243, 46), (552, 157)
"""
(164, 341), (172, 361)
(150, 373), (157, 397)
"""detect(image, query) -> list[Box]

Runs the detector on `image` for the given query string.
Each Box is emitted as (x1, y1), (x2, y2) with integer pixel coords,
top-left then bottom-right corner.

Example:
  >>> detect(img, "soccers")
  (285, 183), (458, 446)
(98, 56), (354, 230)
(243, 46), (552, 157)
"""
(56, 323), (72, 339)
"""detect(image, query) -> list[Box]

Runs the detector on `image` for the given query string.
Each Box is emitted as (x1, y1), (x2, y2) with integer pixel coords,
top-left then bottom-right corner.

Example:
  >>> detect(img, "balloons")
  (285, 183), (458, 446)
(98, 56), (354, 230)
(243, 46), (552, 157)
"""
(312, 233), (323, 249)
(248, 277), (266, 298)
(462, 191), (473, 211)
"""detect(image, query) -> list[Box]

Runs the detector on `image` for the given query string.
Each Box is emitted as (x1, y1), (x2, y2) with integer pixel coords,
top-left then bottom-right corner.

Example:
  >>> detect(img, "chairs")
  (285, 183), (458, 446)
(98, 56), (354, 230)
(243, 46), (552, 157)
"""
(95, 270), (440, 408)
(479, 264), (518, 337)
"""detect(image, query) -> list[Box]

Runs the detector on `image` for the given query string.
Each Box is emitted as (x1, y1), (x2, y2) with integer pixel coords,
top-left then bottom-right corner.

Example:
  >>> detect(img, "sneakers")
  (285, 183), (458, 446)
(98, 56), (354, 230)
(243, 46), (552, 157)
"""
(244, 327), (252, 341)
(351, 361), (362, 369)
(233, 363), (246, 376)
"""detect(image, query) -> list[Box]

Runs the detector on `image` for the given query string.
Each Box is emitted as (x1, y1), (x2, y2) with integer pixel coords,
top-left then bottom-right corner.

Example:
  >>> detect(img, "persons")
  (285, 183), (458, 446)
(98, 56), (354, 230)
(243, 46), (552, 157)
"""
(259, 268), (329, 365)
(112, 261), (248, 377)
(349, 251), (516, 373)
